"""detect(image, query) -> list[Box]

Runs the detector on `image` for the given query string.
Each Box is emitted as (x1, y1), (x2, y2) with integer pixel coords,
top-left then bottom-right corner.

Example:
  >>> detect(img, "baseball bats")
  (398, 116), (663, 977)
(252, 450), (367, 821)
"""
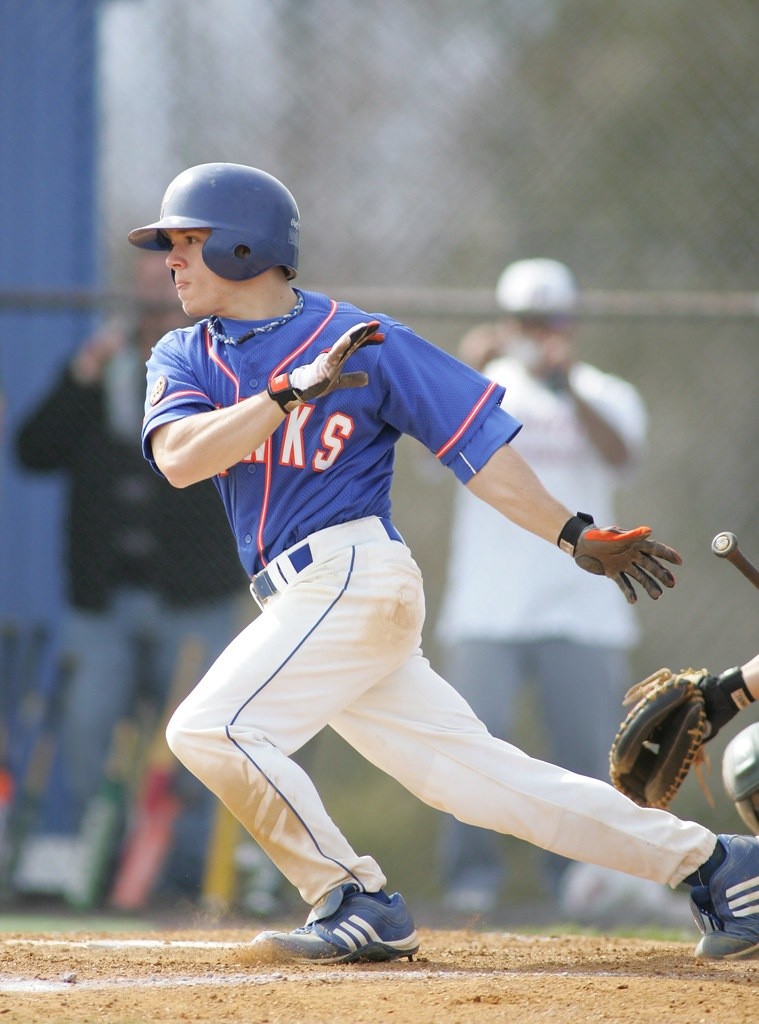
(709, 531), (759, 590)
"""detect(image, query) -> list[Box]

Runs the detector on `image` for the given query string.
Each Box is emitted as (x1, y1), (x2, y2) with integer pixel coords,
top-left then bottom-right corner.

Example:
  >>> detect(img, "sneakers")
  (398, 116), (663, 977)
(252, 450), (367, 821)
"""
(689, 835), (759, 960)
(249, 883), (419, 963)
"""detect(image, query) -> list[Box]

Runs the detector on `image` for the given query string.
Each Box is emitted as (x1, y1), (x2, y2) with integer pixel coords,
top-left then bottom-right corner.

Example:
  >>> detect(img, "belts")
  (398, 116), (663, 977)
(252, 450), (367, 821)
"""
(253, 518), (403, 601)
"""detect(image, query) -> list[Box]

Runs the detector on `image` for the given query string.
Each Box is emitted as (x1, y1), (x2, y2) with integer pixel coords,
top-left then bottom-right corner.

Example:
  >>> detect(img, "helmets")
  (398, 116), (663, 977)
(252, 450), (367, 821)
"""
(128, 163), (299, 281)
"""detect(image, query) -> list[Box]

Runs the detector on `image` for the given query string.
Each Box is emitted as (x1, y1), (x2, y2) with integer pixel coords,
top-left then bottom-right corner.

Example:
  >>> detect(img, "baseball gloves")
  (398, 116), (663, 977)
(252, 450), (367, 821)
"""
(608, 665), (758, 811)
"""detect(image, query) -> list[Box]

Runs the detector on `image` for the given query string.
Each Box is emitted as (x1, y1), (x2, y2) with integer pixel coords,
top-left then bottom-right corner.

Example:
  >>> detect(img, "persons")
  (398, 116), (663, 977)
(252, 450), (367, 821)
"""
(15, 248), (292, 921)
(128, 161), (759, 966)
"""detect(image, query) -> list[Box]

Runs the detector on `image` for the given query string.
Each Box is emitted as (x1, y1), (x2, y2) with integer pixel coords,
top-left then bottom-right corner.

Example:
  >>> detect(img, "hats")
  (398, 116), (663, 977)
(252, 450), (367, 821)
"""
(494, 257), (574, 313)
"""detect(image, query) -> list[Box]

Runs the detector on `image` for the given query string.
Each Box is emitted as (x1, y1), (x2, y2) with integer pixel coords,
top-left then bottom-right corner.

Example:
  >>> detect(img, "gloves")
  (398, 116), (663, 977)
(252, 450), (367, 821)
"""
(557, 511), (682, 605)
(267, 321), (385, 414)
